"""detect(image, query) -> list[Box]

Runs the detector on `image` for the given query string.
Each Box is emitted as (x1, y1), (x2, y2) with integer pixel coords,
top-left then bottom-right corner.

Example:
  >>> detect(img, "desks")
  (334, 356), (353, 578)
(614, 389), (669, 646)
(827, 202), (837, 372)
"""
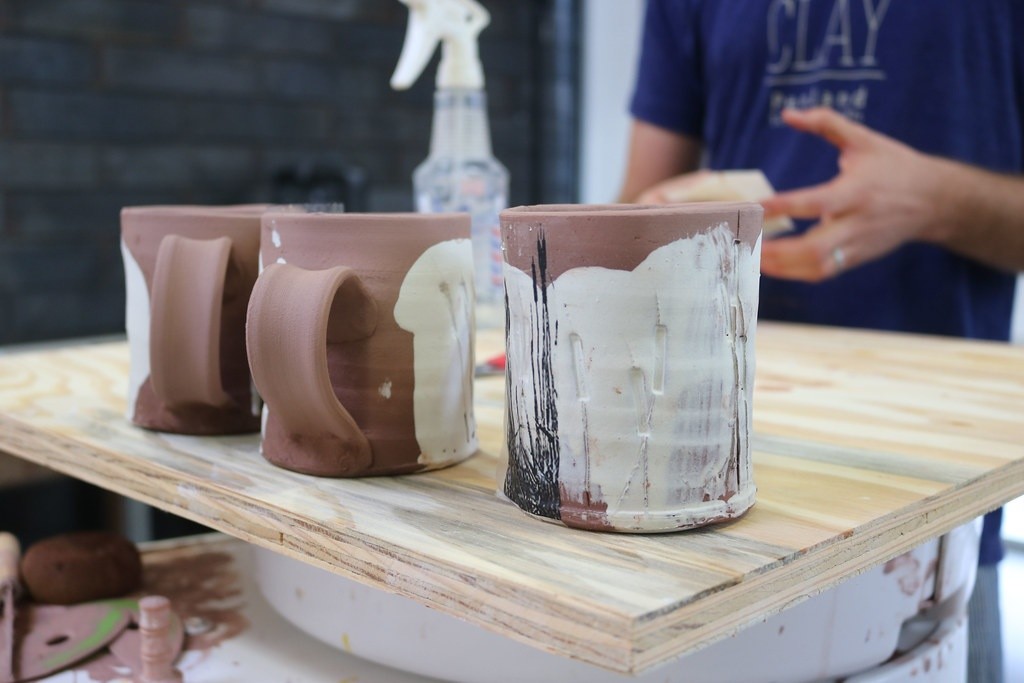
(0, 325), (1024, 683)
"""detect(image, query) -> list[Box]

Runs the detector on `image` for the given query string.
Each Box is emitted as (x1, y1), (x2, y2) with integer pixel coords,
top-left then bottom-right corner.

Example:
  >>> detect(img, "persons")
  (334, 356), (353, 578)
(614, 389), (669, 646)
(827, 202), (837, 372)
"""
(620, 0), (1024, 683)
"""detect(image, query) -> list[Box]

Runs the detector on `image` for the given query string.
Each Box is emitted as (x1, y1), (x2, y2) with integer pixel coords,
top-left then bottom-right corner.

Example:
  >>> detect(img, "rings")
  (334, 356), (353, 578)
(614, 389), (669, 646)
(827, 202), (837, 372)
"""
(832, 249), (844, 272)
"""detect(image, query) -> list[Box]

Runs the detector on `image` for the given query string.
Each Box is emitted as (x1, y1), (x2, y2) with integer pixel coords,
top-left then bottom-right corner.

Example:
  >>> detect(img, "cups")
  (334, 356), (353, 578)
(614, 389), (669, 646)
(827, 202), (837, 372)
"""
(120, 204), (277, 436)
(499, 201), (775, 533)
(245, 209), (480, 477)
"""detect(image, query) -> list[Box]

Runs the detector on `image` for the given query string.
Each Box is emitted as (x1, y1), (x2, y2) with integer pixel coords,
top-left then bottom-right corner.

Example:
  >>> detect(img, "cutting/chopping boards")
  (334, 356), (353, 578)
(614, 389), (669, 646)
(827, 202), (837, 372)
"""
(0, 308), (1024, 677)
